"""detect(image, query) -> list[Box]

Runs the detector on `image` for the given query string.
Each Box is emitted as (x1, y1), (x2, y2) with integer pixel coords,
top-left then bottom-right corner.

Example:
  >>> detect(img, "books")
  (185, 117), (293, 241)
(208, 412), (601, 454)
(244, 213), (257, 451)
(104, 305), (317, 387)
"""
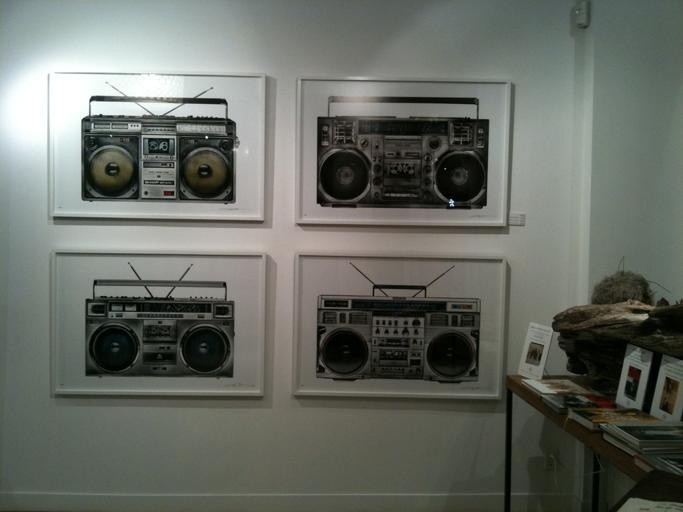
(521, 376), (682, 476)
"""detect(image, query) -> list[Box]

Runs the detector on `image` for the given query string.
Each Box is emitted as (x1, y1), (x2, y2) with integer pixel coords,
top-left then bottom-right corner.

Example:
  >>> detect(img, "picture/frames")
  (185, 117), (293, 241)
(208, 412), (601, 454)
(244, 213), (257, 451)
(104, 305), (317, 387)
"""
(49, 247), (271, 402)
(290, 249), (509, 403)
(293, 73), (515, 232)
(46, 64), (268, 225)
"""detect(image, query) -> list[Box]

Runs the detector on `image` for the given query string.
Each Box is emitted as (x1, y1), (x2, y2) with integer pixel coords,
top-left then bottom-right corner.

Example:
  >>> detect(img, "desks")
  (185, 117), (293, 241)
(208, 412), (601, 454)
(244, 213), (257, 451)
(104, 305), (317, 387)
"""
(503, 374), (683, 511)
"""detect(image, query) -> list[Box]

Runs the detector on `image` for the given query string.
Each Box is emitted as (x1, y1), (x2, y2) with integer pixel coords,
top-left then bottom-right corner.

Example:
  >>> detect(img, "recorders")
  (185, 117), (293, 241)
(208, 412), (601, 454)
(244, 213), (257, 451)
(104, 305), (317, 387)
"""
(77, 79), (240, 206)
(315, 261), (482, 385)
(83, 261), (236, 380)
(316, 96), (489, 211)
